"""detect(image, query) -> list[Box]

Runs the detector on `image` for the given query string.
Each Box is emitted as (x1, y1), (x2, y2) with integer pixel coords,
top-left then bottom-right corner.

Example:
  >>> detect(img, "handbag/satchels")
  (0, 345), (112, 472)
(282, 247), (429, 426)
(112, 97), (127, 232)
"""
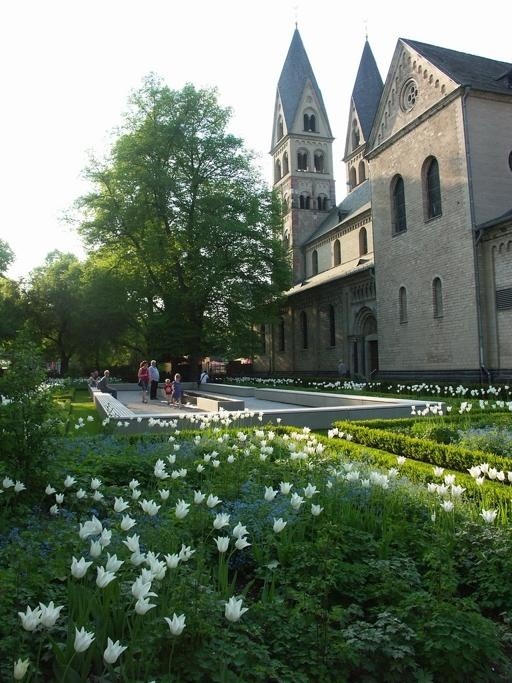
(138, 379), (146, 386)
(196, 379), (201, 384)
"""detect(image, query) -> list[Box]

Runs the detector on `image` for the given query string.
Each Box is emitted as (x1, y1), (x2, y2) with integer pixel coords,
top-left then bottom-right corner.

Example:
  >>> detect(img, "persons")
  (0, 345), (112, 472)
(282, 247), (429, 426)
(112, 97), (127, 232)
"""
(199, 368), (210, 383)
(148, 358), (160, 400)
(94, 367), (102, 382)
(163, 379), (174, 406)
(170, 371), (183, 405)
(97, 369), (117, 399)
(137, 360), (149, 403)
(337, 358), (346, 378)
(89, 371), (99, 387)
